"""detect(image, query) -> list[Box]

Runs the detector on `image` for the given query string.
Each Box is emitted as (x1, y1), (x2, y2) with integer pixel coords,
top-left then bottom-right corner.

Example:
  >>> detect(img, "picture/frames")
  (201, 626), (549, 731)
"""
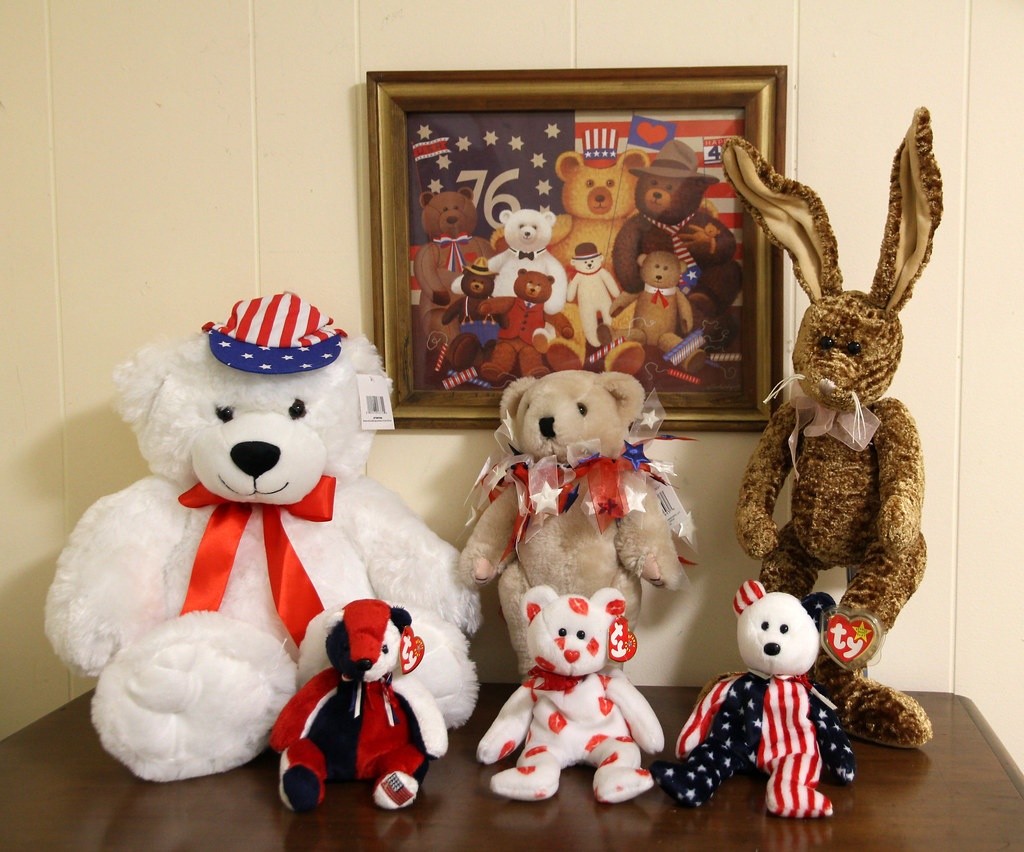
(367, 64), (789, 433)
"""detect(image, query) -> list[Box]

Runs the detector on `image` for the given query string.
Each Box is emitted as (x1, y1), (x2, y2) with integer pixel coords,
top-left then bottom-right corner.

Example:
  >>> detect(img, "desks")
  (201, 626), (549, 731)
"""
(0, 684), (1024, 852)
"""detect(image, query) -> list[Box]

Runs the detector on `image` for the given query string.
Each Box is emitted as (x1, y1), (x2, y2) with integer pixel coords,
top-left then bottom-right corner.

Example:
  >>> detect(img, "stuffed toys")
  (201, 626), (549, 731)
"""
(476, 585), (665, 803)
(44, 291), (482, 782)
(650, 581), (856, 818)
(459, 371), (699, 688)
(271, 599), (449, 811)
(721, 108), (944, 748)
